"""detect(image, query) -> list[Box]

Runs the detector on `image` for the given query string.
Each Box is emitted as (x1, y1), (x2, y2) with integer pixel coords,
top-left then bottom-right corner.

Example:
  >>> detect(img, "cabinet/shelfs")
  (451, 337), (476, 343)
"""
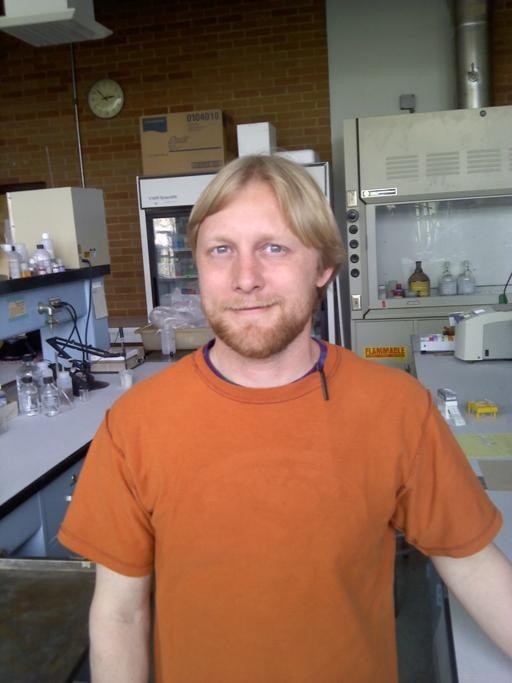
(0, 454), (84, 536)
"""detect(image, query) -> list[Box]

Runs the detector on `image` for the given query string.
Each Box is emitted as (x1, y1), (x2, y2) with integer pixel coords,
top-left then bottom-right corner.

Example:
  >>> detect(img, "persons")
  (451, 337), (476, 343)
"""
(55, 150), (512, 683)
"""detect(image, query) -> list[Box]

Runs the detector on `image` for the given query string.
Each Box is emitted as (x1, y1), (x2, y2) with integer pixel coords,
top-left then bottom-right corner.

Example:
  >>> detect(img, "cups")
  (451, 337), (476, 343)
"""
(120, 370), (133, 388)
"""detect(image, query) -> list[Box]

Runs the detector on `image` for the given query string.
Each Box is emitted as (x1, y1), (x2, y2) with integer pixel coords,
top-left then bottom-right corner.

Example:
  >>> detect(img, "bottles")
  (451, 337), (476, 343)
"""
(378, 260), (475, 299)
(15, 355), (86, 417)
(167, 352), (176, 365)
(0, 233), (66, 281)
(161, 323), (176, 355)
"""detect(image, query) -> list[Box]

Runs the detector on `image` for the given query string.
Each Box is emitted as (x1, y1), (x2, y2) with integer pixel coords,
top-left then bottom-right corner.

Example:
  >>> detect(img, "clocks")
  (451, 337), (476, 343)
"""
(87, 77), (126, 119)
